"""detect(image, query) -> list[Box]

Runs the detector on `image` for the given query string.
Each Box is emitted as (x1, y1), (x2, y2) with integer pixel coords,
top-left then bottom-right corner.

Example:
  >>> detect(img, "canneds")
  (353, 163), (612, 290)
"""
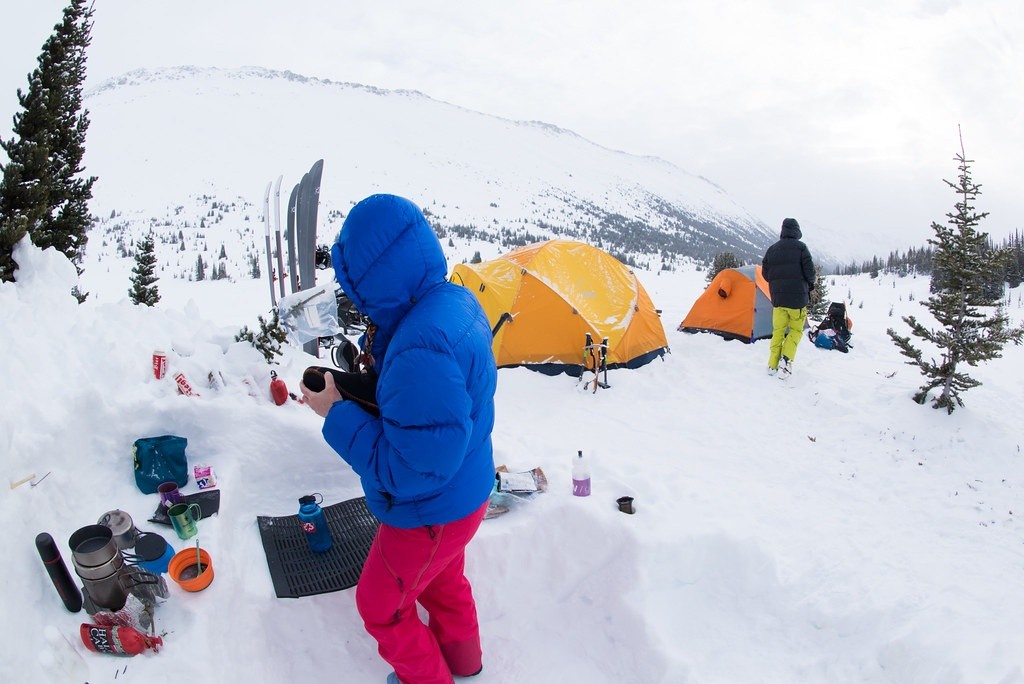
(242, 377), (260, 396)
(172, 370), (201, 397)
(208, 370), (226, 390)
(151, 351), (166, 379)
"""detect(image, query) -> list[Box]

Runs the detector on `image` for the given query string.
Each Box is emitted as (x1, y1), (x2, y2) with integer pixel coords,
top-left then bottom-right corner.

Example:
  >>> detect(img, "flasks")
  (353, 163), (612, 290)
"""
(35, 532), (82, 613)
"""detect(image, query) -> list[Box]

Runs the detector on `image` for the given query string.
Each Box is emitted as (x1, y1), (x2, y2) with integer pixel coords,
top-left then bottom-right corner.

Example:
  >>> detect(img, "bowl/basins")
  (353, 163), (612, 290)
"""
(168, 547), (214, 592)
(96, 510), (139, 550)
(68, 526), (116, 568)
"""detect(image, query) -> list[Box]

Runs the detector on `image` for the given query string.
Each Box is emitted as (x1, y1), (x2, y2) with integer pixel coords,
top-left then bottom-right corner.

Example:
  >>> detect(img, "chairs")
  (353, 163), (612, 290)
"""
(808, 302), (855, 350)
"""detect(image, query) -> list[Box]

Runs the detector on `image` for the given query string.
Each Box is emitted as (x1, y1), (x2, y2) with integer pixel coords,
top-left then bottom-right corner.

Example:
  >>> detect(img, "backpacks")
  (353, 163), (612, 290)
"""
(808, 302), (853, 353)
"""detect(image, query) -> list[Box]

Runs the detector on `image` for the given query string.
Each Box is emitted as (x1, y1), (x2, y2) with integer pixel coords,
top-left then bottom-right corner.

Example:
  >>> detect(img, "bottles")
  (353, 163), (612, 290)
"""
(80, 624), (163, 657)
(571, 451), (591, 500)
(289, 393), (304, 404)
(298, 493), (333, 553)
(269, 370), (288, 406)
(134, 533), (175, 576)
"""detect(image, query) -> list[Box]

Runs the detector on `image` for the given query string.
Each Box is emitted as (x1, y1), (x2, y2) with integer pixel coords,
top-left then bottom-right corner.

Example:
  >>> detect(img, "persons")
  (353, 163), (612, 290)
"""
(297, 193), (498, 684)
(762, 217), (817, 380)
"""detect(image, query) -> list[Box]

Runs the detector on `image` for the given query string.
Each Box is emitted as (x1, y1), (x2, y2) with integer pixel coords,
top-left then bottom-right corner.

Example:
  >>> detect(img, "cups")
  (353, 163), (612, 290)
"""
(167, 503), (201, 541)
(616, 496), (634, 515)
(153, 352), (166, 380)
(172, 369), (200, 397)
(158, 482), (181, 511)
(208, 369), (225, 390)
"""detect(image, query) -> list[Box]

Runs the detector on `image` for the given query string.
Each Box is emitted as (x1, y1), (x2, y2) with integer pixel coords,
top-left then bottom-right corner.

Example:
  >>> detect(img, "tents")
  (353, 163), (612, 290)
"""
(677, 265), (810, 344)
(447, 238), (669, 379)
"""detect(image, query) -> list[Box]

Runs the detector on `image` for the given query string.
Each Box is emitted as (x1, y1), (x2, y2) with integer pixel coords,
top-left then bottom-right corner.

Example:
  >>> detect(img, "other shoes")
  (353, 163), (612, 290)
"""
(387, 672), (402, 684)
(768, 367), (778, 376)
(778, 355), (792, 375)
